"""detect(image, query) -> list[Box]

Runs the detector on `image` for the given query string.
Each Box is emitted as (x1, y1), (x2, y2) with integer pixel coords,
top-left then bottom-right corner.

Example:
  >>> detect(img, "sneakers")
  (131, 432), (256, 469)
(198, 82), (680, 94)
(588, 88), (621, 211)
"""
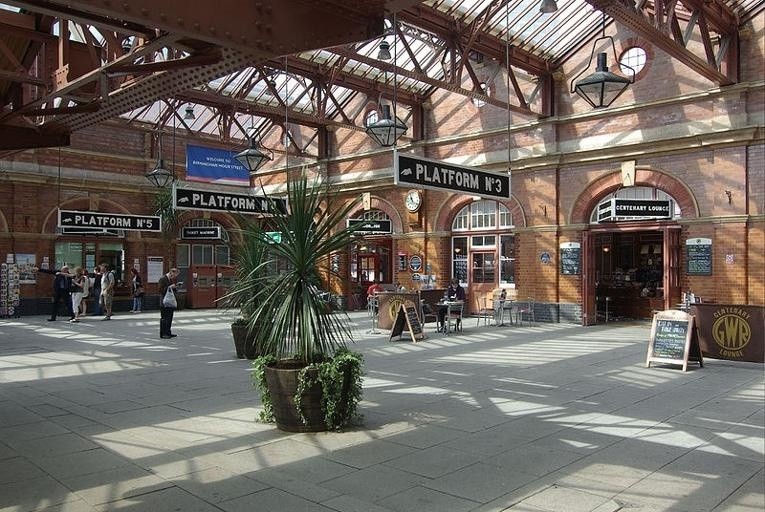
(47, 310), (142, 323)
(454, 327), (460, 331)
(435, 327), (447, 333)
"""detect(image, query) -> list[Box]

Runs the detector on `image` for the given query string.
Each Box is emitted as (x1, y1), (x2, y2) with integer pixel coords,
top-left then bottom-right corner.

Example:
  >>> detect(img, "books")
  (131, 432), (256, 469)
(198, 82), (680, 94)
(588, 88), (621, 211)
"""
(175, 281), (184, 286)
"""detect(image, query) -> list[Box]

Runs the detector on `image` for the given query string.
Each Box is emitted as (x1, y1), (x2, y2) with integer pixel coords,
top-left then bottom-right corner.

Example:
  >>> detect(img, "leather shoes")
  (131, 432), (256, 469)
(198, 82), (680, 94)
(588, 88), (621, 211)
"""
(160, 334), (177, 339)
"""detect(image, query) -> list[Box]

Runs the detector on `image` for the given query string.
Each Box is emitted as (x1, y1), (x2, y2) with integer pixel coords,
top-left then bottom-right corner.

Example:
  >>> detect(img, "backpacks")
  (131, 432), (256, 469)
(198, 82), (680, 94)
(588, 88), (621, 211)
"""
(107, 272), (121, 285)
(80, 276), (89, 297)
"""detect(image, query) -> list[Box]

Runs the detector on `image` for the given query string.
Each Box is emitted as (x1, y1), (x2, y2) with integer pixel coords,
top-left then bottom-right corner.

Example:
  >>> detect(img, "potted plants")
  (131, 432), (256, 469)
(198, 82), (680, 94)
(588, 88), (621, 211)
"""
(214, 165), (380, 433)
(214, 210), (280, 359)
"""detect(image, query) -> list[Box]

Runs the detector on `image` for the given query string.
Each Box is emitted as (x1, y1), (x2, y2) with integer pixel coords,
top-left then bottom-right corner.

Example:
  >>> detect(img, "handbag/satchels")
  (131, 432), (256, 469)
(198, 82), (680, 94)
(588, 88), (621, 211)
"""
(135, 287), (145, 297)
(163, 290), (177, 308)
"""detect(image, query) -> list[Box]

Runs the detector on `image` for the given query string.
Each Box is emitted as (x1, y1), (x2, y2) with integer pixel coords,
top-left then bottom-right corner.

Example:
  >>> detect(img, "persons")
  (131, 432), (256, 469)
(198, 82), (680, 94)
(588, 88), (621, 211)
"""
(394, 280), (407, 293)
(46, 263), (115, 322)
(129, 268), (142, 314)
(366, 279), (385, 315)
(158, 267), (181, 339)
(435, 278), (465, 333)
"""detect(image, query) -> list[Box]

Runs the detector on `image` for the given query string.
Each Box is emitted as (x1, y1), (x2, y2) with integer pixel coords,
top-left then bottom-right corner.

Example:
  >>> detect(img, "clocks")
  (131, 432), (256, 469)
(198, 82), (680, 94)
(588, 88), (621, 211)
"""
(404, 189), (422, 213)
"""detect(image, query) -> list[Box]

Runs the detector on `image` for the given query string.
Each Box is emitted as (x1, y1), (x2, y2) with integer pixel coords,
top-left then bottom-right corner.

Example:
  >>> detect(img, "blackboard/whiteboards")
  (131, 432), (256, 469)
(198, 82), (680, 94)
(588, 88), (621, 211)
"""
(402, 302), (423, 338)
(647, 310), (694, 366)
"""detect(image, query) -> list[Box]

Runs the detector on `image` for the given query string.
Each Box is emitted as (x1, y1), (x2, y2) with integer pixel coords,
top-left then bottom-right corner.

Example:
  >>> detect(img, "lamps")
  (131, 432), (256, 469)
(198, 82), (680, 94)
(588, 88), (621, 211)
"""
(233, 115), (273, 171)
(364, 70), (408, 146)
(142, 100), (177, 188)
(570, 7), (635, 109)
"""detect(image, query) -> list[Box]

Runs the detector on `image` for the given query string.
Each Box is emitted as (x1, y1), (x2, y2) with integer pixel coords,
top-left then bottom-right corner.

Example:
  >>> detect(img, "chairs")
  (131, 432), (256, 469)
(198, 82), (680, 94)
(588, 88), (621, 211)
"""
(421, 297), (535, 336)
